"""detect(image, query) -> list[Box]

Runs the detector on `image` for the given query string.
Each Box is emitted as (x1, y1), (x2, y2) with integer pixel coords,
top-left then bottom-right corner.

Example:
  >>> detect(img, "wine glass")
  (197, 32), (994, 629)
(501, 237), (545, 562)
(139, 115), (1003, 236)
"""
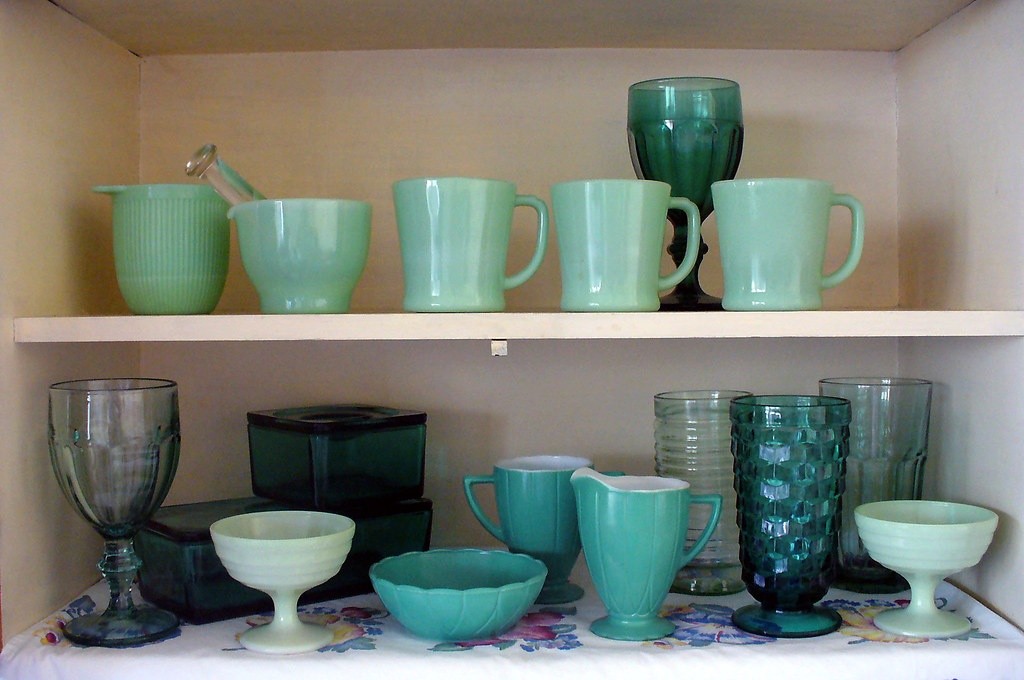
(626, 76), (745, 311)
(209, 510), (358, 655)
(43, 376), (183, 646)
(853, 500), (1000, 639)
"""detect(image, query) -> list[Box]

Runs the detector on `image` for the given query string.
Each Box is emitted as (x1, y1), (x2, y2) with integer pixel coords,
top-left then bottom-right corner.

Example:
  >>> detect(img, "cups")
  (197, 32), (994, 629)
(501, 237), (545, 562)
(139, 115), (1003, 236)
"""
(391, 176), (550, 311)
(652, 388), (754, 596)
(727, 394), (853, 639)
(709, 177), (865, 310)
(226, 197), (374, 314)
(570, 466), (724, 642)
(460, 454), (596, 605)
(553, 180), (702, 312)
(91, 181), (232, 315)
(818, 375), (934, 595)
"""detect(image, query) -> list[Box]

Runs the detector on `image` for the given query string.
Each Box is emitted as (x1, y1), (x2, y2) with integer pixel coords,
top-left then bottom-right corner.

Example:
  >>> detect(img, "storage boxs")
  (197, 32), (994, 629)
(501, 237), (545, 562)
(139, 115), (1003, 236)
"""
(129, 495), (435, 626)
(246, 404), (428, 508)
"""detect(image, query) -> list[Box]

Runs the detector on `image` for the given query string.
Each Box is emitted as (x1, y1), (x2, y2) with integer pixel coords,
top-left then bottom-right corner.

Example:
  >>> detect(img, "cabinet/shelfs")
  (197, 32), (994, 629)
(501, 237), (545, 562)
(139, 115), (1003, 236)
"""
(1, 0), (1021, 680)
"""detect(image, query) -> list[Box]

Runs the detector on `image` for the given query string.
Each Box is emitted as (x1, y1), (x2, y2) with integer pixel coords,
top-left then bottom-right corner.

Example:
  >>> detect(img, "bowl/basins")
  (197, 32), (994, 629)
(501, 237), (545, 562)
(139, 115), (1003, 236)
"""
(368, 548), (548, 641)
